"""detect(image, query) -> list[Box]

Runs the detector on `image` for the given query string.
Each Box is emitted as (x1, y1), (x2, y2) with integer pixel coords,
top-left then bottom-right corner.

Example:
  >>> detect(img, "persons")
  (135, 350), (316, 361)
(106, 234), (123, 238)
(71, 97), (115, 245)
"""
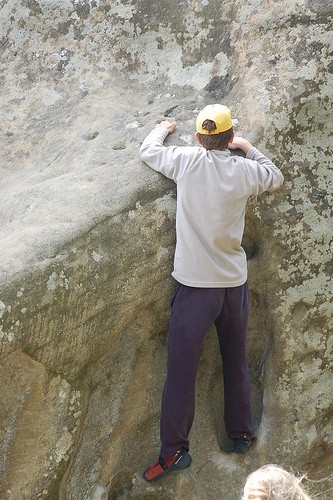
(242, 463), (311, 500)
(139, 104), (284, 482)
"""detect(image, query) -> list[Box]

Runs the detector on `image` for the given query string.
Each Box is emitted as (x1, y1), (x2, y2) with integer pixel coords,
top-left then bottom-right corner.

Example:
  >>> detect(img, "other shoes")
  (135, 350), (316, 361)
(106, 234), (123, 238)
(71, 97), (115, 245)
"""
(232, 433), (255, 452)
(142, 449), (193, 483)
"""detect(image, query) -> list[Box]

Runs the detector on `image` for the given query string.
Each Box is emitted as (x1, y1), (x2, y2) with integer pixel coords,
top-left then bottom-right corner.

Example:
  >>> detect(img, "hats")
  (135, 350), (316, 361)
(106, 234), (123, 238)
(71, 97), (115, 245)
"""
(196, 103), (236, 135)
